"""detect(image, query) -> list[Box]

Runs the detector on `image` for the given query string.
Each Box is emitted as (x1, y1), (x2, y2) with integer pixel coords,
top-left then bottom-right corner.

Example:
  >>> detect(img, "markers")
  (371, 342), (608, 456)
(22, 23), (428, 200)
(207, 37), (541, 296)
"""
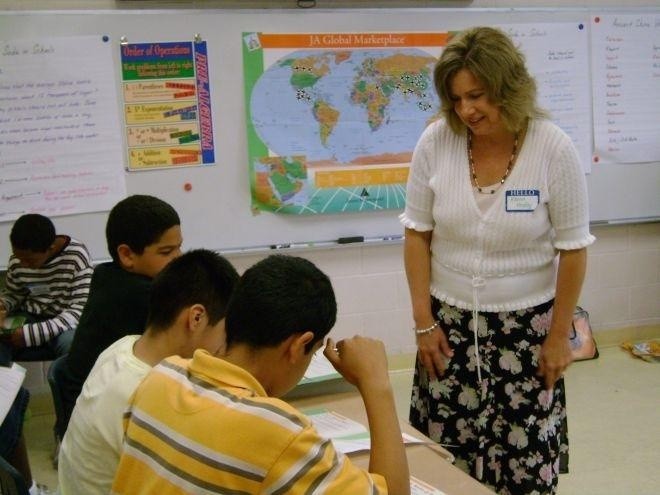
(271, 244), (294, 249)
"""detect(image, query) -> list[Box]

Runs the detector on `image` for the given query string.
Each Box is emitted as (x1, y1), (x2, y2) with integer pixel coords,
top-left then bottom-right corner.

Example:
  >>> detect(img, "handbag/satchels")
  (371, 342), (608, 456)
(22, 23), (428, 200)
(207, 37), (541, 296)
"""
(569, 305), (599, 361)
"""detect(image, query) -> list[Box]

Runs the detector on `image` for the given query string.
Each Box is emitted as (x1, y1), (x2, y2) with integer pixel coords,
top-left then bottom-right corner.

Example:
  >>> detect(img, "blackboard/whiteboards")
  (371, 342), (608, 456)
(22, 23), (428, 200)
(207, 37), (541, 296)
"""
(0, 8), (660, 272)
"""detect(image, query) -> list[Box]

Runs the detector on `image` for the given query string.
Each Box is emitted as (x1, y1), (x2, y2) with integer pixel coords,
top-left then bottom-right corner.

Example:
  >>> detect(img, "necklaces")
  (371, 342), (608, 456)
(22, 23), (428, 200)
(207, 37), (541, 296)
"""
(468, 130), (520, 195)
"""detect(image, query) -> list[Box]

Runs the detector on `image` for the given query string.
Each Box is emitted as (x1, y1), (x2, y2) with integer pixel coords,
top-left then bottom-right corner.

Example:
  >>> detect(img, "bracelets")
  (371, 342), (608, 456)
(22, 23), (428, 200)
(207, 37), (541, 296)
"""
(415, 321), (440, 334)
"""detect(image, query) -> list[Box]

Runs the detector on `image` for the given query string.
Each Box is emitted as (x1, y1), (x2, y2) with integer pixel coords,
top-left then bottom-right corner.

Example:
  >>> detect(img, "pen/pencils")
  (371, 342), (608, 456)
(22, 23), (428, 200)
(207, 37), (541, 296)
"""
(589, 220), (609, 224)
(384, 235), (402, 240)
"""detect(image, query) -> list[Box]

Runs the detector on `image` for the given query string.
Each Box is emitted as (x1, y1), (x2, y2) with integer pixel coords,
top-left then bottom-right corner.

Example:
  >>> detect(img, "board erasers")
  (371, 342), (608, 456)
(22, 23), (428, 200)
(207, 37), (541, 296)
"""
(338, 237), (363, 244)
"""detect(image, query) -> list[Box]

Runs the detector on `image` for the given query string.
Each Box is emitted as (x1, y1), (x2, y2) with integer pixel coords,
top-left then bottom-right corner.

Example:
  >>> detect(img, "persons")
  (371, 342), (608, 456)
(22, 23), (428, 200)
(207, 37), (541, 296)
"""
(0, 214), (94, 367)
(54, 196), (184, 469)
(110, 253), (411, 495)
(1, 360), (54, 494)
(400, 27), (596, 495)
(58, 248), (240, 495)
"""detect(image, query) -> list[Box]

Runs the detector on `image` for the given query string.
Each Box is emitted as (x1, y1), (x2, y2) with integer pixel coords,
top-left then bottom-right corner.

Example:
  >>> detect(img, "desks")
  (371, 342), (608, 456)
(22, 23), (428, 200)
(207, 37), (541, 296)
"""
(283, 393), (501, 495)
